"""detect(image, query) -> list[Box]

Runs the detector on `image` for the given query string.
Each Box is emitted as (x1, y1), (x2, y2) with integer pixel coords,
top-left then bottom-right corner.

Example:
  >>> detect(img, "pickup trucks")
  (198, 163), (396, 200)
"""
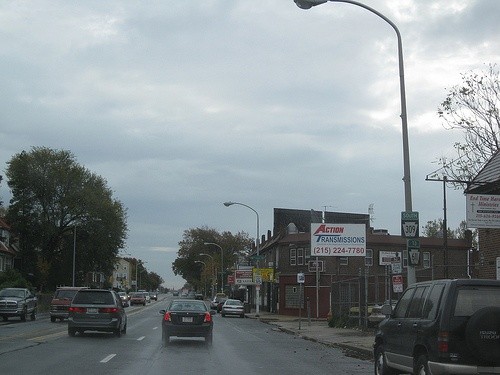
(0, 288), (37, 321)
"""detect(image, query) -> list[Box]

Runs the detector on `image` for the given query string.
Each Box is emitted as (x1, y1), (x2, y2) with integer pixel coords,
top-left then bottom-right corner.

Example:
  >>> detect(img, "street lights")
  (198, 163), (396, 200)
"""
(224, 201), (261, 317)
(200, 253), (215, 300)
(204, 242), (224, 294)
(72, 218), (102, 286)
(194, 261), (207, 299)
(293, 0), (417, 287)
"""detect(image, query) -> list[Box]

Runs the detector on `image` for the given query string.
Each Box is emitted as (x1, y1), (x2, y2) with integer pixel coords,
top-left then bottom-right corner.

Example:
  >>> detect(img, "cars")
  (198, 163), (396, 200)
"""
(173, 291), (179, 297)
(367, 299), (398, 328)
(216, 298), (229, 314)
(194, 294), (204, 300)
(221, 299), (248, 319)
(130, 293), (146, 306)
(117, 291), (131, 307)
(159, 299), (216, 346)
(181, 292), (188, 297)
(128, 292), (136, 300)
(138, 290), (160, 304)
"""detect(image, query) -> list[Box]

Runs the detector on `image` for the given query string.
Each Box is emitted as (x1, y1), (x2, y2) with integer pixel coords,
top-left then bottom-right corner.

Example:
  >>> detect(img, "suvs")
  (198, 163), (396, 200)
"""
(373, 278), (500, 375)
(49, 287), (91, 323)
(67, 289), (129, 337)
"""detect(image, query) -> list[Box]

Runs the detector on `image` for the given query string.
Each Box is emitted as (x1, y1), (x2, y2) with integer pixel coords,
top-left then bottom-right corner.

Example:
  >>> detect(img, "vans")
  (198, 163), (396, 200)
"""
(210, 293), (228, 310)
